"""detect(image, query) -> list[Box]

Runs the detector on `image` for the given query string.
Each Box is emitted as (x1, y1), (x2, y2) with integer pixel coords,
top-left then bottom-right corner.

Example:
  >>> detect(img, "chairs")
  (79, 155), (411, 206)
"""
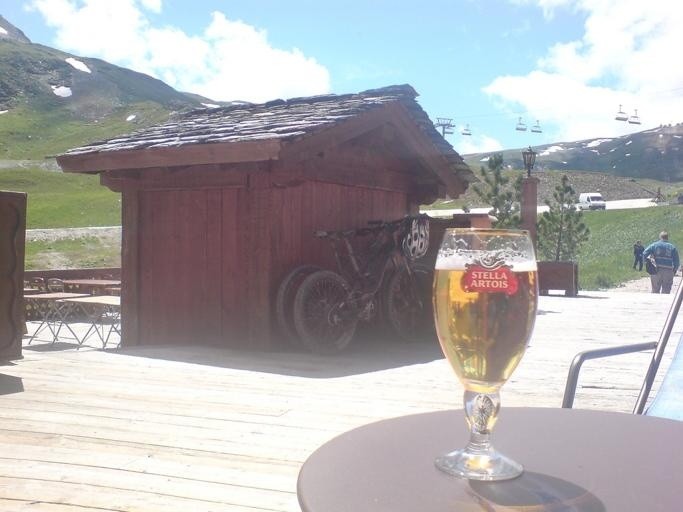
(561, 275), (683, 421)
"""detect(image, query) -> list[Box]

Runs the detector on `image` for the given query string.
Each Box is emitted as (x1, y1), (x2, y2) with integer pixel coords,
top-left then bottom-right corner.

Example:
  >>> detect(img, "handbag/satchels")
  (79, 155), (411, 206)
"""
(646, 254), (657, 275)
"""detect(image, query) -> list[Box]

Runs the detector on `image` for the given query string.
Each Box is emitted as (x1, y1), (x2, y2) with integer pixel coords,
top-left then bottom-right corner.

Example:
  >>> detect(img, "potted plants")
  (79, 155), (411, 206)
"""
(535, 175), (590, 297)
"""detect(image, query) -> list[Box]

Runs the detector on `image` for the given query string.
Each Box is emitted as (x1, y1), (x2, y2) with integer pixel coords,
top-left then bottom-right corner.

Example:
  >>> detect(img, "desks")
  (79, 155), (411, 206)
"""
(295, 405), (683, 512)
(24, 272), (121, 351)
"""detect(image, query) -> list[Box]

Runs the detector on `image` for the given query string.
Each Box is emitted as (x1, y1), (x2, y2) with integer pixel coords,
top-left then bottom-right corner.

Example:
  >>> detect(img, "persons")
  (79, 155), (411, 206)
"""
(631, 240), (645, 271)
(643, 231), (680, 294)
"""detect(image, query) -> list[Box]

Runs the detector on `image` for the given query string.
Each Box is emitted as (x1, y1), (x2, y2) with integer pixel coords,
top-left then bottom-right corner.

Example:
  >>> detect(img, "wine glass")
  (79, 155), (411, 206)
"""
(432, 228), (540, 481)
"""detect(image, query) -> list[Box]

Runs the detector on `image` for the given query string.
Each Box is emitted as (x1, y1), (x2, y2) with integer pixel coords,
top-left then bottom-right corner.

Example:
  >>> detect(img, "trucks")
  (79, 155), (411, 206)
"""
(577, 191), (606, 212)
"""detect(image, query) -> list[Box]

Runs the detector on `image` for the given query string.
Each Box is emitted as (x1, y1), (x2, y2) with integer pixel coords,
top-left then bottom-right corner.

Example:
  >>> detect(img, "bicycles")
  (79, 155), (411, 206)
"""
(272, 211), (435, 355)
